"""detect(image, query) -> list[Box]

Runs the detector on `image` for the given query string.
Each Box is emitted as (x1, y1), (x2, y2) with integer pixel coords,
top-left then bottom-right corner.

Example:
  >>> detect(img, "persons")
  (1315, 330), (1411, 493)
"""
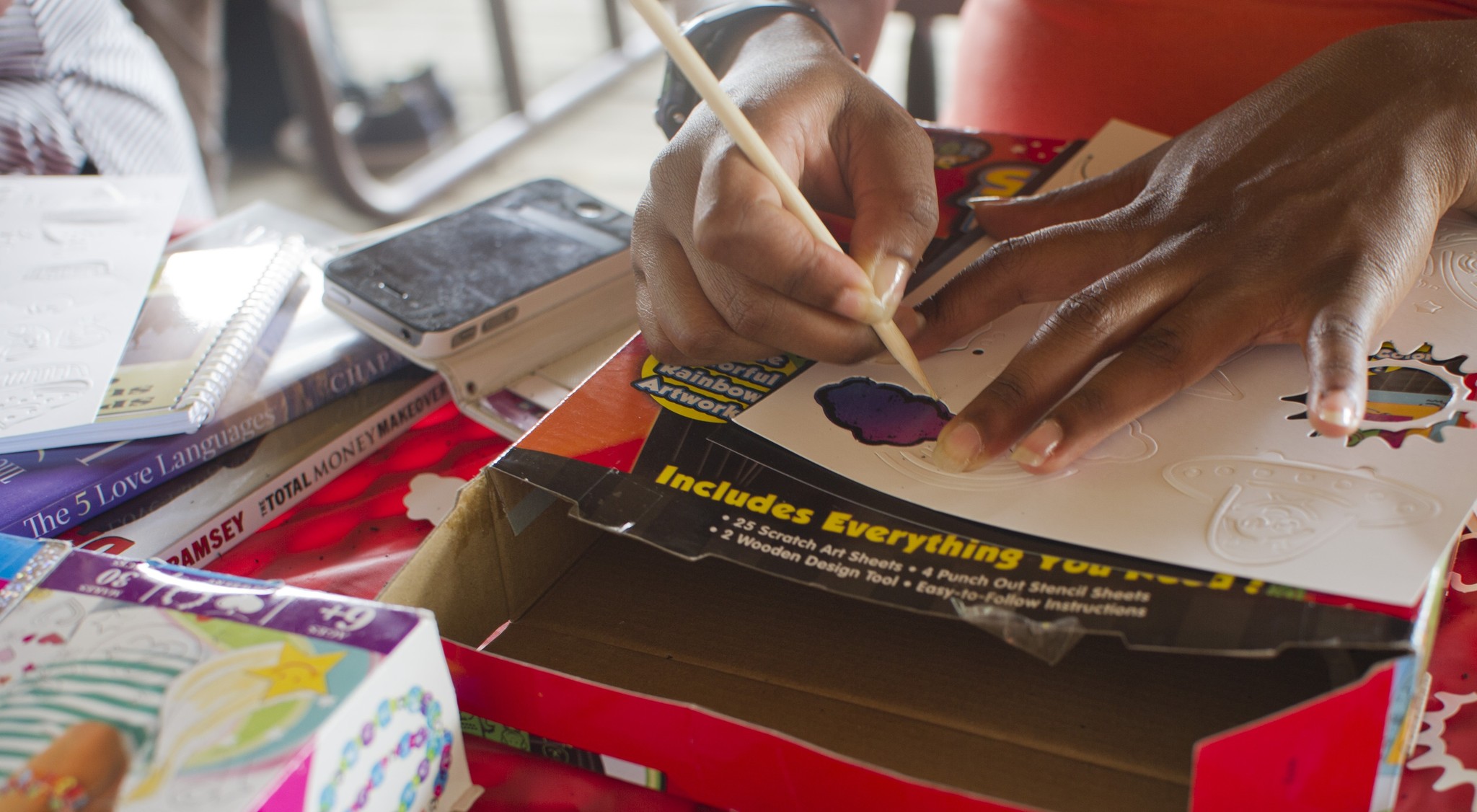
(625, 0), (1476, 480)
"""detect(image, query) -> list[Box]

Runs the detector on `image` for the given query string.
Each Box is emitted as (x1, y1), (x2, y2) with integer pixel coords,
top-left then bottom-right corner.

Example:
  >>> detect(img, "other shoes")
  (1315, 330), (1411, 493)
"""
(278, 67), (460, 165)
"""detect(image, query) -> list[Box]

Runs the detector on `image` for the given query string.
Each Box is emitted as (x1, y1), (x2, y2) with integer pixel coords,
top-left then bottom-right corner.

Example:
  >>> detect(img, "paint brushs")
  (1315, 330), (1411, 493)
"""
(630, 0), (940, 403)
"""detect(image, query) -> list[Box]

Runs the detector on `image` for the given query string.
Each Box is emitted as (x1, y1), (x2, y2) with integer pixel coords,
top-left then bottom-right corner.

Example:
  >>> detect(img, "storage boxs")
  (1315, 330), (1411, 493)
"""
(0, 115), (1462, 811)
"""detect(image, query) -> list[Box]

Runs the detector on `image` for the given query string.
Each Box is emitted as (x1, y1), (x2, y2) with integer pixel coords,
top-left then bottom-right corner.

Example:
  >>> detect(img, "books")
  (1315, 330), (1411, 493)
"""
(1, 197), (445, 571)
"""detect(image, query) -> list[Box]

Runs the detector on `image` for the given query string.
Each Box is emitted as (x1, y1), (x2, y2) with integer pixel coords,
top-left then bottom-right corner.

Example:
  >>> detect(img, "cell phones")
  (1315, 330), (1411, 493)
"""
(321, 173), (634, 360)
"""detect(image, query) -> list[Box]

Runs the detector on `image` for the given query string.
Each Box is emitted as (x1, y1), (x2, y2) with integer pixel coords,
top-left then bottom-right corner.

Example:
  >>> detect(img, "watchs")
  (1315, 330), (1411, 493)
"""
(655, 1), (862, 142)
